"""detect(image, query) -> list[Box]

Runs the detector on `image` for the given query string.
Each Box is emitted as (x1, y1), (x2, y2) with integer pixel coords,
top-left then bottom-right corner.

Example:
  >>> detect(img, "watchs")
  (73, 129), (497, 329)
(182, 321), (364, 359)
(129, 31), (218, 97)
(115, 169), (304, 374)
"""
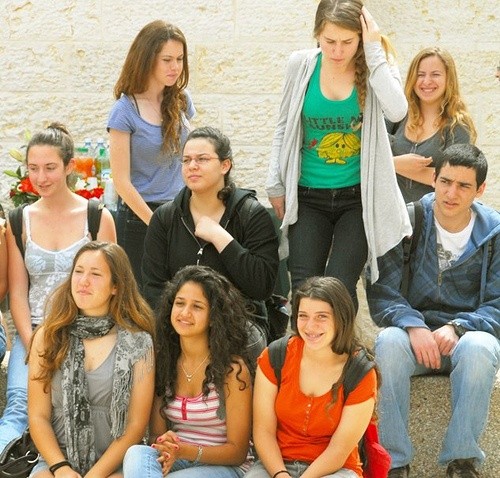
(448, 319), (465, 336)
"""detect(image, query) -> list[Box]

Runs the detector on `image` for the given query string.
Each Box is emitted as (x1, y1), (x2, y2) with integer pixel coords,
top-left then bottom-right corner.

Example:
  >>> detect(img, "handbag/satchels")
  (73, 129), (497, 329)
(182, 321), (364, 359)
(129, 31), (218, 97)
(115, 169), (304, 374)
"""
(1, 425), (42, 478)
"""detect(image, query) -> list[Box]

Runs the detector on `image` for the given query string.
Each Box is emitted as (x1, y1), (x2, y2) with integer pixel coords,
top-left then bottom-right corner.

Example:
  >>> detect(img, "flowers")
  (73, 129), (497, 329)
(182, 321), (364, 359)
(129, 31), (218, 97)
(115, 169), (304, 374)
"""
(3, 148), (105, 207)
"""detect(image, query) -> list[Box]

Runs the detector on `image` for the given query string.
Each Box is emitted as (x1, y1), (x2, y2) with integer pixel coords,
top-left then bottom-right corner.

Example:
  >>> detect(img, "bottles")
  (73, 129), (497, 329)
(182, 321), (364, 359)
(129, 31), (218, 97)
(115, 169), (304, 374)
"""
(94, 142), (108, 158)
(75, 147), (93, 177)
(94, 148), (111, 188)
(83, 140), (94, 157)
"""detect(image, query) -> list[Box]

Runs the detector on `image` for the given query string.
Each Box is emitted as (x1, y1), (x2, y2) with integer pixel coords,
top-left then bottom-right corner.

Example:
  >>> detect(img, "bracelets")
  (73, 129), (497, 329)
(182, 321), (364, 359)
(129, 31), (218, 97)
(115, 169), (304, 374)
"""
(188, 446), (203, 465)
(49, 461), (72, 475)
(273, 470), (291, 478)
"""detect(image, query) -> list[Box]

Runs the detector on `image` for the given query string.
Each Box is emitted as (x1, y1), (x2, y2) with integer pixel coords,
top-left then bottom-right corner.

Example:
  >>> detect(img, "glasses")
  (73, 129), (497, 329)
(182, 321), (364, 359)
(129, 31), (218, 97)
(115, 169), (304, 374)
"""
(178, 156), (221, 165)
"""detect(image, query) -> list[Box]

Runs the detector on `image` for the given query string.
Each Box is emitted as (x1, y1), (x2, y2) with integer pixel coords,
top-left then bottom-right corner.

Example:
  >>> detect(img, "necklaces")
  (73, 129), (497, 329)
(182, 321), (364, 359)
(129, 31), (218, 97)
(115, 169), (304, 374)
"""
(180, 351), (210, 383)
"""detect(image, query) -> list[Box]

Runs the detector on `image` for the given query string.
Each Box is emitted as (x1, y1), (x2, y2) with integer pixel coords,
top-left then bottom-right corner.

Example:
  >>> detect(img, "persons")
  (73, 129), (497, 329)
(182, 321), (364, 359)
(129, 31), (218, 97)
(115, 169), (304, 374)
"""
(0, 218), (10, 361)
(122, 265), (253, 477)
(240, 276), (376, 478)
(107, 21), (196, 293)
(266, 0), (414, 334)
(381, 45), (478, 205)
(0, 121), (116, 457)
(142, 126), (279, 343)
(364, 142), (498, 478)
(24, 240), (156, 477)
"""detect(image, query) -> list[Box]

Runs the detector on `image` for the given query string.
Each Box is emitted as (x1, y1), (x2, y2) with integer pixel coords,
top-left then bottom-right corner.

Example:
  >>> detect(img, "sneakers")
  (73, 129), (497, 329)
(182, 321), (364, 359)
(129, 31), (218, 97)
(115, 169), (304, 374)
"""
(447, 460), (479, 478)
(388, 464), (410, 478)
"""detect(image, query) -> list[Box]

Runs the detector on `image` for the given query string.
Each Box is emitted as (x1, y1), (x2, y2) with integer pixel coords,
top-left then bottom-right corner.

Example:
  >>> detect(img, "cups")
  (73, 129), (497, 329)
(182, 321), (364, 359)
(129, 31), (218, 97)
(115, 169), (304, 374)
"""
(103, 181), (118, 211)
